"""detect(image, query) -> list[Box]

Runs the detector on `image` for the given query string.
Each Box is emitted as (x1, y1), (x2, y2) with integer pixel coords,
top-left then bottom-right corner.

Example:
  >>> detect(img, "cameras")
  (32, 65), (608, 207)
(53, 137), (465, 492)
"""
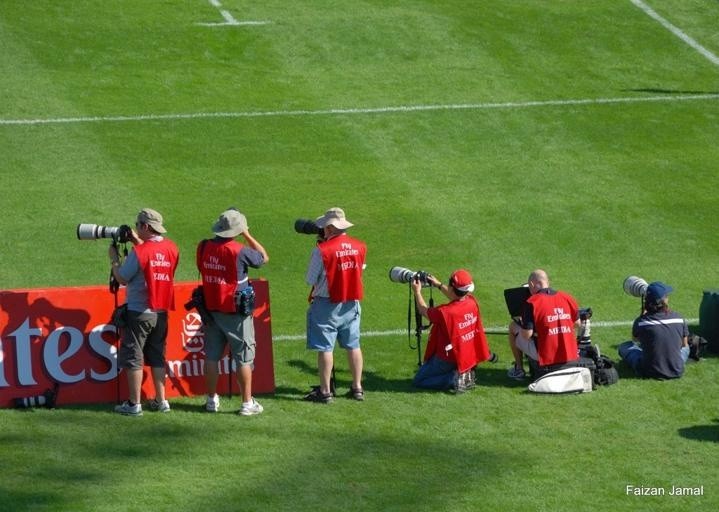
(184, 284), (214, 325)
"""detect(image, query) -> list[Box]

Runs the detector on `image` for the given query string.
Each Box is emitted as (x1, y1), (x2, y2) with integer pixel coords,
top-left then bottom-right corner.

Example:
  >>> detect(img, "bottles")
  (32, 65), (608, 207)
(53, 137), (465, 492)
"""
(242, 286), (255, 312)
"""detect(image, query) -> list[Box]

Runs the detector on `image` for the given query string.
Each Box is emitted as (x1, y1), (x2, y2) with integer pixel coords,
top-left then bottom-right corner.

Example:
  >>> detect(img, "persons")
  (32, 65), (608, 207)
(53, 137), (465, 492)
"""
(507, 269), (580, 382)
(411, 270), (490, 395)
(618, 281), (691, 380)
(196, 210), (269, 415)
(109, 207), (179, 417)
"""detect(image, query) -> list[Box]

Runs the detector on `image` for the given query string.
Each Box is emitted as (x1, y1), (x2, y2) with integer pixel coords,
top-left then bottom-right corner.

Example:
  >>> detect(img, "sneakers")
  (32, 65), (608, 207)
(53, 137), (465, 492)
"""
(508, 366), (526, 380)
(149, 399), (170, 413)
(114, 401), (143, 417)
(205, 393), (220, 412)
(239, 399), (263, 415)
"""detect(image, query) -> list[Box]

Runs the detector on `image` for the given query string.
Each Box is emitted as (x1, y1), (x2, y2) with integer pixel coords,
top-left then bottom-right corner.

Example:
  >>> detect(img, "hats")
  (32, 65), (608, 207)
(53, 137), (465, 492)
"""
(450, 269), (473, 292)
(316, 207), (353, 230)
(645, 281), (673, 300)
(212, 209), (249, 238)
(137, 207), (168, 234)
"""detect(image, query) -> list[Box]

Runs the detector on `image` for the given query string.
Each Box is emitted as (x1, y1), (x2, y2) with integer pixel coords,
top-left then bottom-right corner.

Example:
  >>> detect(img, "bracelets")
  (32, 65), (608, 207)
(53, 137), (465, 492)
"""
(439, 283), (443, 289)
(111, 255), (118, 264)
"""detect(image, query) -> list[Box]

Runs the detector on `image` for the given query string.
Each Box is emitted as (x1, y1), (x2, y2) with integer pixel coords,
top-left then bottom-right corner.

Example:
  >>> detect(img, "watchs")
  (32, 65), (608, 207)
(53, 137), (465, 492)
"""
(303, 206), (368, 404)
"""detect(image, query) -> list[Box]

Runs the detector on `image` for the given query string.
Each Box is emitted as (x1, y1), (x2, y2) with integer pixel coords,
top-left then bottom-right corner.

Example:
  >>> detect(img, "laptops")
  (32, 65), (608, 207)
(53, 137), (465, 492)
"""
(504, 287), (532, 325)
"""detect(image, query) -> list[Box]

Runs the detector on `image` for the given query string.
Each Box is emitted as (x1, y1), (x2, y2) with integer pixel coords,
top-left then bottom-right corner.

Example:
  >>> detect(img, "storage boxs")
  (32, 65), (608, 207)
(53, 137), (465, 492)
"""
(529, 355), (599, 393)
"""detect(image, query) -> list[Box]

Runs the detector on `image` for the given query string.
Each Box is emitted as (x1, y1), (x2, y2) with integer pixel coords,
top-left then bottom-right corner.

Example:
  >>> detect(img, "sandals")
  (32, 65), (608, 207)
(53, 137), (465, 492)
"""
(348, 386), (366, 400)
(303, 390), (332, 403)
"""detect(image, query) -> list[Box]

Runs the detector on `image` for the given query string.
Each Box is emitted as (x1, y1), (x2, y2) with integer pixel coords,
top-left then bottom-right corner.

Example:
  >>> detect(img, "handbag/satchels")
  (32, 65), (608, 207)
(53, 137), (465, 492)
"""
(112, 303), (130, 329)
(234, 285), (254, 314)
